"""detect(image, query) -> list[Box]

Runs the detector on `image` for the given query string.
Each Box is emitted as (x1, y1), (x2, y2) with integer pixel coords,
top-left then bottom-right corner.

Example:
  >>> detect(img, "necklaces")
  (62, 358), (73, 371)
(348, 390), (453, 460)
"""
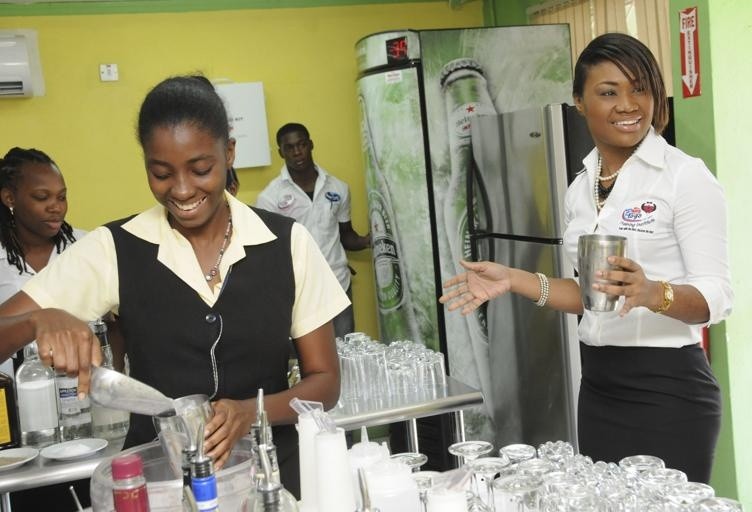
(167, 199), (232, 280)
(594, 141), (644, 211)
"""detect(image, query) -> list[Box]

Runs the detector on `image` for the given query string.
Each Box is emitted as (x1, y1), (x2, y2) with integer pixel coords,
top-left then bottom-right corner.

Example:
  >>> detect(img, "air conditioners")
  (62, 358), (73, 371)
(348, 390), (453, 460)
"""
(0, 35), (34, 99)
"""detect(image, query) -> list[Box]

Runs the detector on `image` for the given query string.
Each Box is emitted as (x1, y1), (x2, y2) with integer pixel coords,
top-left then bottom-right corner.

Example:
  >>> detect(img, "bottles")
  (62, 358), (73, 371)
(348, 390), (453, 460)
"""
(0, 319), (128, 453)
(108, 388), (390, 512)
(355, 94), (422, 342)
(436, 57), (501, 412)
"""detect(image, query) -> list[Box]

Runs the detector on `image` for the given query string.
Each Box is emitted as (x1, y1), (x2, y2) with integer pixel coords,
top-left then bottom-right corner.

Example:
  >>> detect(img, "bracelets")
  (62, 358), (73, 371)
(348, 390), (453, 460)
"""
(532, 271), (549, 307)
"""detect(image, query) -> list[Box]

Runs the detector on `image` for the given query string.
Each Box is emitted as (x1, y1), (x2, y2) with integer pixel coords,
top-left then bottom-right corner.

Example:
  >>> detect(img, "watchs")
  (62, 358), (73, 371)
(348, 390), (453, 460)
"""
(652, 280), (675, 314)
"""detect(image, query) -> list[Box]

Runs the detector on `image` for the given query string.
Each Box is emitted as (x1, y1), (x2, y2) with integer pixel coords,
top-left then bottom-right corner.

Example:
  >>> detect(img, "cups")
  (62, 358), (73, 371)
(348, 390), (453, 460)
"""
(334, 331), (447, 413)
(577, 233), (629, 314)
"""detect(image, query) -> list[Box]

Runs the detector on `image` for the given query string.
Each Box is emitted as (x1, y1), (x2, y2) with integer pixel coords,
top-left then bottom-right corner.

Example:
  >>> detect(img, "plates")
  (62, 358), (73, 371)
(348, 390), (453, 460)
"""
(40, 436), (109, 463)
(0, 446), (40, 472)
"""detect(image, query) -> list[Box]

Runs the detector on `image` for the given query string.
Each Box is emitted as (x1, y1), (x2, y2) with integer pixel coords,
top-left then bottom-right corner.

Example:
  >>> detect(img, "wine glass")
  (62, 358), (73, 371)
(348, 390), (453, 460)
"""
(390, 436), (741, 512)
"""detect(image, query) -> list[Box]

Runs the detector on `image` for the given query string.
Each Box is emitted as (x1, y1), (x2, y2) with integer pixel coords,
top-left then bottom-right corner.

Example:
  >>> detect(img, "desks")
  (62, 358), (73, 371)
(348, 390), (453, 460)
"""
(1, 373), (485, 511)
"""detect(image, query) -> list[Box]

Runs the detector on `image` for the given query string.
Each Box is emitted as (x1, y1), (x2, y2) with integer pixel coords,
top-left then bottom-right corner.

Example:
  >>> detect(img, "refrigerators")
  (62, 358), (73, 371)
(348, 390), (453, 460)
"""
(349, 23), (579, 472)
(463, 95), (677, 457)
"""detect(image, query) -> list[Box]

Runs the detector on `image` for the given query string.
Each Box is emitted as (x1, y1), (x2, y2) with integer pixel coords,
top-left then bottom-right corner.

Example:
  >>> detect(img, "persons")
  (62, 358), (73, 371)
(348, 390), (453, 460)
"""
(256, 123), (371, 335)
(0, 146), (127, 375)
(434, 29), (727, 485)
(0, 65), (355, 470)
